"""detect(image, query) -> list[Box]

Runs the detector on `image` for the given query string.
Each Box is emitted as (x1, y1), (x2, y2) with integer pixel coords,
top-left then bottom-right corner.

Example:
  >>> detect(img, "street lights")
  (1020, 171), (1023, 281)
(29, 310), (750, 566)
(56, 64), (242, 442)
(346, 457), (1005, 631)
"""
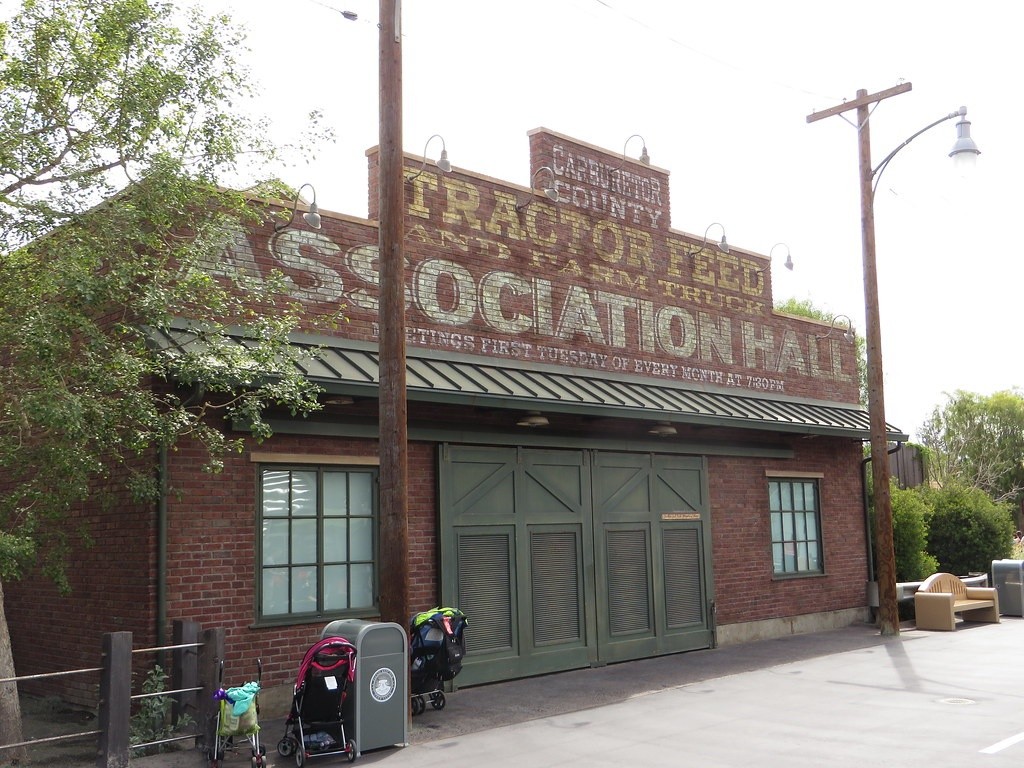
(858, 106), (983, 635)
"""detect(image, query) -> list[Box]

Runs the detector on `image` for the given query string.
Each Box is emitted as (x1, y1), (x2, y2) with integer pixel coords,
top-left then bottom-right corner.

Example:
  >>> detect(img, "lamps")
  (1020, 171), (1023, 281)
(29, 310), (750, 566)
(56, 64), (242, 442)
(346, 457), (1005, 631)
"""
(609, 134), (650, 175)
(758, 242), (793, 278)
(275, 182), (321, 233)
(689, 222), (729, 258)
(817, 314), (854, 345)
(648, 421), (677, 438)
(516, 166), (559, 212)
(407, 135), (453, 184)
(325, 394), (354, 412)
(516, 410), (549, 429)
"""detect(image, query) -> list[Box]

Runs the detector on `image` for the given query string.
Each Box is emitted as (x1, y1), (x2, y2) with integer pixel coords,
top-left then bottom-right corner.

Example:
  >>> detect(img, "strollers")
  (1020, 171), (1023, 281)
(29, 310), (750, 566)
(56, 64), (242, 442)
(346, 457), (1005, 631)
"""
(277, 636), (361, 768)
(210, 658), (269, 768)
(405, 607), (469, 714)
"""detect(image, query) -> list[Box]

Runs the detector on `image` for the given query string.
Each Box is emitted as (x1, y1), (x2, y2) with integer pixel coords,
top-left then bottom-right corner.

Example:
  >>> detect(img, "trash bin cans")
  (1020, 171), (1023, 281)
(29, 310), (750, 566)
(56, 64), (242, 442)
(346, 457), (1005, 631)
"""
(320, 618), (409, 758)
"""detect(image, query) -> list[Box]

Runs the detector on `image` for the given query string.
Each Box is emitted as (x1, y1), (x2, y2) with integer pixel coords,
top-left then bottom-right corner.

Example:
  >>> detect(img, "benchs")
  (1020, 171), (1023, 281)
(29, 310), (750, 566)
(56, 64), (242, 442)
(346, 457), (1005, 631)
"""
(915, 571), (1000, 632)
